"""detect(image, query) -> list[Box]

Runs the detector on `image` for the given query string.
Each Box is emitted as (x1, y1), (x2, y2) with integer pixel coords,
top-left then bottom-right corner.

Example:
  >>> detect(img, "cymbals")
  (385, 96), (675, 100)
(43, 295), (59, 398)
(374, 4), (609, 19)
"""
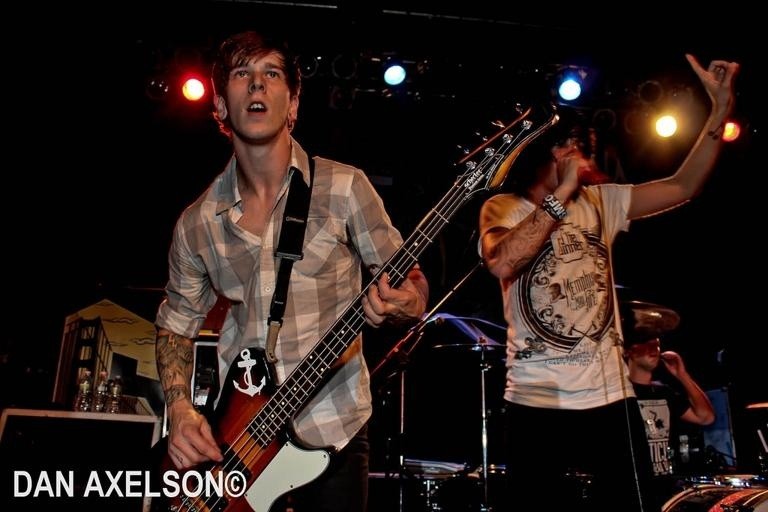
(747, 402), (768, 410)
(432, 336), (510, 358)
(619, 300), (680, 347)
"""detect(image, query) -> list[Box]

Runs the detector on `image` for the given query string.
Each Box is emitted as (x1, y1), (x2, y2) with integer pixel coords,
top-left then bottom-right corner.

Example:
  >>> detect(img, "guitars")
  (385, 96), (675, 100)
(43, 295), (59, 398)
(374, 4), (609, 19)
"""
(119, 95), (558, 512)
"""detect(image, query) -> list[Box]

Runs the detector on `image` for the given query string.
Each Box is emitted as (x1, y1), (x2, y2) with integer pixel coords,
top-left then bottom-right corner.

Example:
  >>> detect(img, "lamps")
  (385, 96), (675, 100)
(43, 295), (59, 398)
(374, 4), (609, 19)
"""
(557, 69), (584, 102)
(378, 52), (409, 88)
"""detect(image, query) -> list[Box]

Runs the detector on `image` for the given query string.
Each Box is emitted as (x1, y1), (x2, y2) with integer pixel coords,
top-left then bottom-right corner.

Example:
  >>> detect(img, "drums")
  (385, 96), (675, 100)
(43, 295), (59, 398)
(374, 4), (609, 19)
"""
(367, 471), (401, 512)
(395, 451), (472, 512)
(662, 476), (767, 512)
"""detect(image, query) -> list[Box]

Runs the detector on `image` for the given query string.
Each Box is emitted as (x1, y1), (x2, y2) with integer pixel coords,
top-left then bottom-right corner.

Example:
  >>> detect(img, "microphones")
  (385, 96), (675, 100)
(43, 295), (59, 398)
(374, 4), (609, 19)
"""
(577, 168), (610, 186)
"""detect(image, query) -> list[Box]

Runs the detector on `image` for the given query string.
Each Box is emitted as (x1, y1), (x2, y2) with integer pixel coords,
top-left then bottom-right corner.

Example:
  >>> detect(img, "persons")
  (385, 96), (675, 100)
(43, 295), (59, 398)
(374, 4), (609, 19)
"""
(622, 333), (716, 511)
(476, 51), (739, 512)
(153, 28), (429, 512)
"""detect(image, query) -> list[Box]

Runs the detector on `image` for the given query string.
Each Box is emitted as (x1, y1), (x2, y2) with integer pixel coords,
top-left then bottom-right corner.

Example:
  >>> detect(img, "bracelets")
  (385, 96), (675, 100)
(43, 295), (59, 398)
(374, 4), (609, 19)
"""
(541, 193), (567, 222)
